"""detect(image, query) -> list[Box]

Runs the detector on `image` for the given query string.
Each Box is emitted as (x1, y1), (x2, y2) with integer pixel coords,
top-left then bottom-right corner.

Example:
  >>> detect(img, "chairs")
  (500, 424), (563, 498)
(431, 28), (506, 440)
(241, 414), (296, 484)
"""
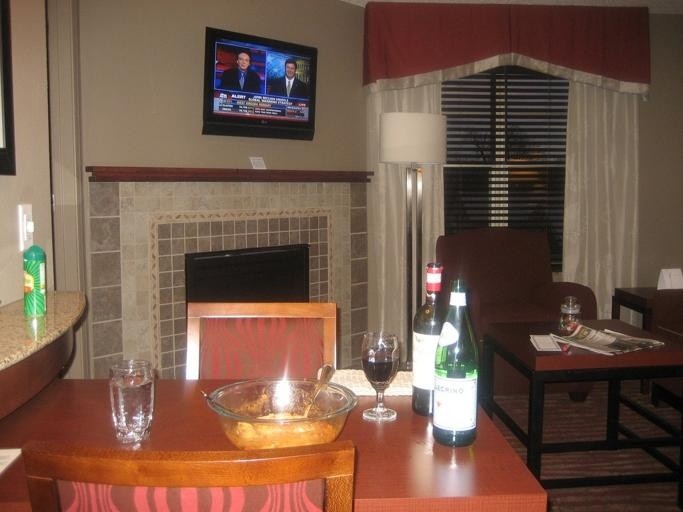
(179, 301), (340, 381)
(20, 434), (358, 508)
(647, 308), (682, 416)
(428, 229), (597, 409)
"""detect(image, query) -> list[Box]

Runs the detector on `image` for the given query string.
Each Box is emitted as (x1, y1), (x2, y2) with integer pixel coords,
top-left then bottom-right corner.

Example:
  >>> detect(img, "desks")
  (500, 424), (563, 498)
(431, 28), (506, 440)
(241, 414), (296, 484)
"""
(610, 282), (682, 393)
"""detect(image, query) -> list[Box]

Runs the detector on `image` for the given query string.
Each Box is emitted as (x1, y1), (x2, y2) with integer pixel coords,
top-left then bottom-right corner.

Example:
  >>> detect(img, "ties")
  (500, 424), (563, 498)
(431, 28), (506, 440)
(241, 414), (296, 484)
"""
(287, 81), (291, 96)
(238, 71), (245, 90)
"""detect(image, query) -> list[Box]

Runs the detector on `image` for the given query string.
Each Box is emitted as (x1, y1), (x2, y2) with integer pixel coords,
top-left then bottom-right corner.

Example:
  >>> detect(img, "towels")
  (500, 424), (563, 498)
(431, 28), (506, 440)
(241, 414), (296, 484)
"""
(316, 365), (418, 399)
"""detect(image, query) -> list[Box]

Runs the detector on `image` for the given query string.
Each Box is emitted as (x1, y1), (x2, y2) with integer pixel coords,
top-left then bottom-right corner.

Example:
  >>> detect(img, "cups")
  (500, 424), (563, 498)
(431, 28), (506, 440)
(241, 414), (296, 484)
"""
(106, 358), (156, 450)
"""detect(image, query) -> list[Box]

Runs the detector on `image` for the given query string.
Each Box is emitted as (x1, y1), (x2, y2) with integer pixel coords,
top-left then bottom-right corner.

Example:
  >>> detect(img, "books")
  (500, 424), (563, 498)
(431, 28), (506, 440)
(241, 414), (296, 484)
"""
(549, 325), (663, 357)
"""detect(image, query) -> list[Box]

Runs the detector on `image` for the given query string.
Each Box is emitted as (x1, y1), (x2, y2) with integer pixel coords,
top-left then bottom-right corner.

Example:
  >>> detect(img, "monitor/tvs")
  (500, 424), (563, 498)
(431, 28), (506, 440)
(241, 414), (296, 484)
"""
(201, 27), (317, 141)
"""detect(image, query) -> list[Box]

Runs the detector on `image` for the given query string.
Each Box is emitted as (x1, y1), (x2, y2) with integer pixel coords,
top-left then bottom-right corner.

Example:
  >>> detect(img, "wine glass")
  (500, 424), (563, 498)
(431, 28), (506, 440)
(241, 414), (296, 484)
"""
(361, 331), (400, 423)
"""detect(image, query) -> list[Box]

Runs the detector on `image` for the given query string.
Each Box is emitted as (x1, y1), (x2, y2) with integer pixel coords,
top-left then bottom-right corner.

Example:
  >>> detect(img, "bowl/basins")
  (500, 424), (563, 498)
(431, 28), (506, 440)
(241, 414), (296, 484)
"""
(205, 376), (358, 452)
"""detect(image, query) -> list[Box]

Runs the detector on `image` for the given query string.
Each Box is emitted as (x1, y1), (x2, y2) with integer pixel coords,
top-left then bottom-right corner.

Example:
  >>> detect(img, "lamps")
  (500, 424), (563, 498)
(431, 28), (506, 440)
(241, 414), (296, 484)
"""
(375, 109), (446, 374)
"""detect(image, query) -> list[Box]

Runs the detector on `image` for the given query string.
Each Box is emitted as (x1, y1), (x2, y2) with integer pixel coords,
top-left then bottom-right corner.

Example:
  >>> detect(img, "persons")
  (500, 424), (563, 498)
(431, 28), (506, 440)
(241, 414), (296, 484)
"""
(219, 51), (259, 93)
(267, 58), (306, 98)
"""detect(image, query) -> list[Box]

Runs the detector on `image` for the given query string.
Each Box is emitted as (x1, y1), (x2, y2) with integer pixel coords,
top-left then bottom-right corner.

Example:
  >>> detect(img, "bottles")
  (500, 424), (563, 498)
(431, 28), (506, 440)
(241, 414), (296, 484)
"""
(21, 245), (45, 318)
(558, 296), (582, 336)
(22, 319), (45, 340)
(411, 262), (479, 447)
